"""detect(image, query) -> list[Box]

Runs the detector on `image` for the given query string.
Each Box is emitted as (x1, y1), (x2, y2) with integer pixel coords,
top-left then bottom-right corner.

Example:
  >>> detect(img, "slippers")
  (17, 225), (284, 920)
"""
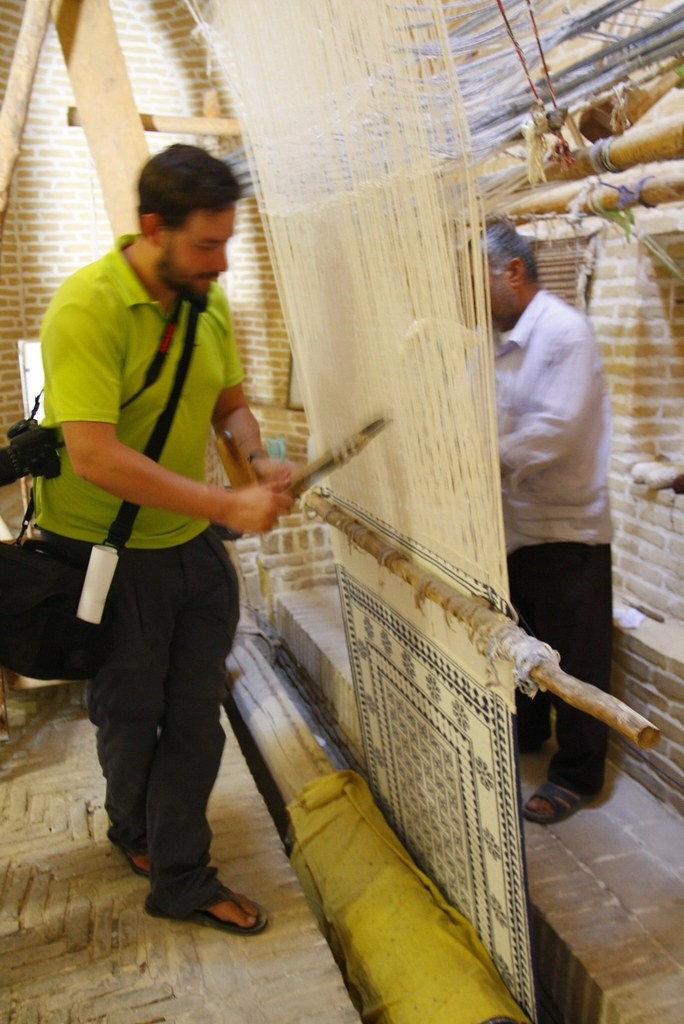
(522, 780), (594, 823)
(107, 825), (153, 879)
(146, 878), (269, 934)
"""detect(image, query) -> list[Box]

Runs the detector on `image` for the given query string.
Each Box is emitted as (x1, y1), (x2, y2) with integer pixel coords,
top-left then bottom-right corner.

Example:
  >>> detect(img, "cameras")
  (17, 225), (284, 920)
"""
(0, 417), (63, 487)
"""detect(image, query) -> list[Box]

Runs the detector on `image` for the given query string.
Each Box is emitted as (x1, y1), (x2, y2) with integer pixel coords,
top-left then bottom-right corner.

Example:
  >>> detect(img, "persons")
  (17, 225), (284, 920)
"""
(33, 141), (298, 937)
(473, 215), (626, 826)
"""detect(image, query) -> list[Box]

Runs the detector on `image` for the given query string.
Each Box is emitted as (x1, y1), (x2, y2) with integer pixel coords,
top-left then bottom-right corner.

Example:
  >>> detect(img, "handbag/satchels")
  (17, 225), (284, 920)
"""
(0, 539), (119, 680)
